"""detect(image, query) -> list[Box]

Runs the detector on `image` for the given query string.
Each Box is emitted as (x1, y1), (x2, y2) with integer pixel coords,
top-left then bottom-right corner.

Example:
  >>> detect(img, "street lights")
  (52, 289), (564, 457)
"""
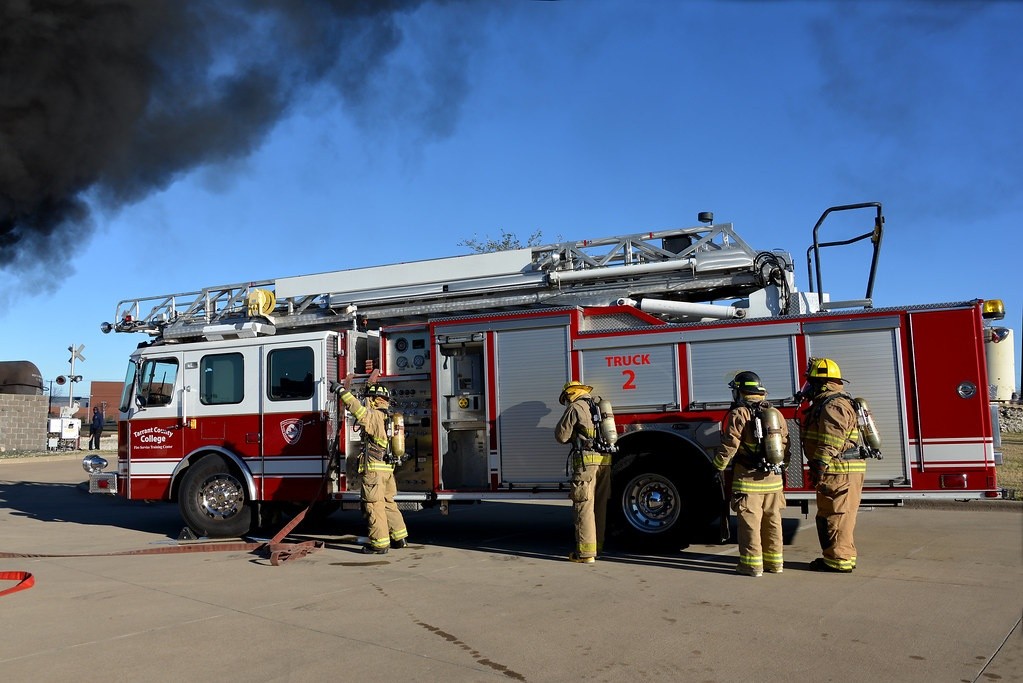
(88, 395), (94, 423)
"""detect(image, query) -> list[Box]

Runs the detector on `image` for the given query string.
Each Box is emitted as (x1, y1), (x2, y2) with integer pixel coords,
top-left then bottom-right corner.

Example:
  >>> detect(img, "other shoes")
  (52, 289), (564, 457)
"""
(569, 551), (595, 563)
(596, 550), (601, 558)
(764, 569), (782, 573)
(363, 545), (388, 554)
(390, 538), (406, 548)
(736, 565), (763, 577)
(808, 558), (856, 573)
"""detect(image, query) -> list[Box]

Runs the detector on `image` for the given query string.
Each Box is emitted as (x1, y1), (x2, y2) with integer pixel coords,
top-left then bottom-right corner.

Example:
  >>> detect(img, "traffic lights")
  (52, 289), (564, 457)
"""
(73, 375), (82, 383)
(56, 375), (66, 385)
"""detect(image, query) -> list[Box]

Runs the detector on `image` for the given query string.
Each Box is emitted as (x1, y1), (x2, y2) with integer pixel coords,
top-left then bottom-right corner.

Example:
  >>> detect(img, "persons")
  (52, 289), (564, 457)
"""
(329, 380), (408, 553)
(92, 406), (104, 449)
(713, 370), (790, 576)
(555, 381), (612, 564)
(802, 357), (866, 573)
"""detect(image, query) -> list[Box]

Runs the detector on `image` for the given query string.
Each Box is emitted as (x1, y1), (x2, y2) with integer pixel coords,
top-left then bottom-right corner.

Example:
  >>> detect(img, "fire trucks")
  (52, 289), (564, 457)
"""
(82, 202), (1016, 551)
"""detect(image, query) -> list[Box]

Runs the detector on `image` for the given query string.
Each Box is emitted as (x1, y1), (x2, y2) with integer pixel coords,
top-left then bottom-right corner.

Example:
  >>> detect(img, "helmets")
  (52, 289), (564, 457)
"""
(363, 383), (390, 401)
(728, 370), (765, 394)
(810, 359), (841, 379)
(559, 381), (593, 406)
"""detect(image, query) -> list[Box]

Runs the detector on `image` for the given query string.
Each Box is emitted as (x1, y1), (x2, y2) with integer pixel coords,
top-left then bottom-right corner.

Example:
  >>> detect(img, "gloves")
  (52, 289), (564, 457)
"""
(807, 459), (826, 487)
(329, 379), (346, 395)
(708, 462), (721, 484)
